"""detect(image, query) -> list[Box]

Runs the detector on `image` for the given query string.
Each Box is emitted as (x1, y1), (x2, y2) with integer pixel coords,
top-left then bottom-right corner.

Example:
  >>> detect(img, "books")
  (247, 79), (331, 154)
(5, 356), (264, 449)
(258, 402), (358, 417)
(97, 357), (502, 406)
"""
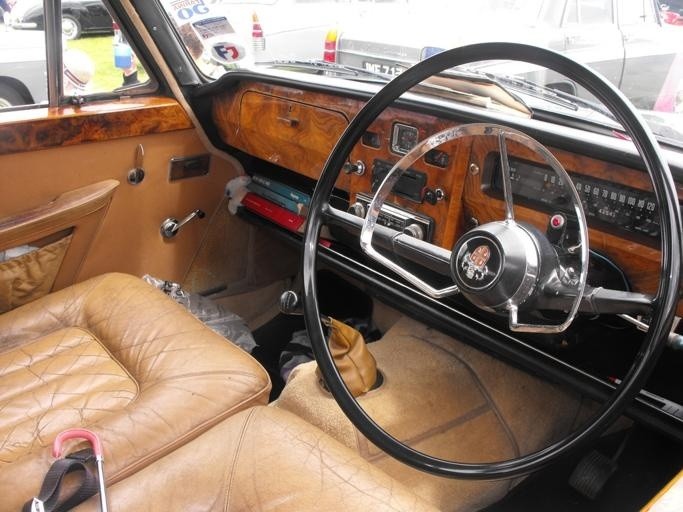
(242, 182), (309, 218)
(250, 173), (312, 206)
(407, 71), (534, 120)
(238, 192), (332, 248)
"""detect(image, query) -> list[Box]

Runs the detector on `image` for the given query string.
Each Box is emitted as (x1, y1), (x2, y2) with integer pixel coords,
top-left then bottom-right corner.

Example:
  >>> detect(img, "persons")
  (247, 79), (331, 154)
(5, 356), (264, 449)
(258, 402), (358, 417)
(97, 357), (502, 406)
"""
(119, 22), (210, 87)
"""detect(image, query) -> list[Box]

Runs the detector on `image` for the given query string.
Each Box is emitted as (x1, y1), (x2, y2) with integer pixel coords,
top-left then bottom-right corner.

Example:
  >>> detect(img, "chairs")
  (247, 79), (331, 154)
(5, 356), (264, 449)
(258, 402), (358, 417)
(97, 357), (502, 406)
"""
(0, 269), (273, 512)
(61, 404), (450, 512)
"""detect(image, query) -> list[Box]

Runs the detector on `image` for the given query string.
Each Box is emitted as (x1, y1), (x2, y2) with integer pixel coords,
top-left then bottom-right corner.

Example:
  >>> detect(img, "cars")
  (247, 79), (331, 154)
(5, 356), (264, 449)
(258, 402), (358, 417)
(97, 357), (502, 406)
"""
(0, 9), (94, 115)
(6, 3), (120, 42)
